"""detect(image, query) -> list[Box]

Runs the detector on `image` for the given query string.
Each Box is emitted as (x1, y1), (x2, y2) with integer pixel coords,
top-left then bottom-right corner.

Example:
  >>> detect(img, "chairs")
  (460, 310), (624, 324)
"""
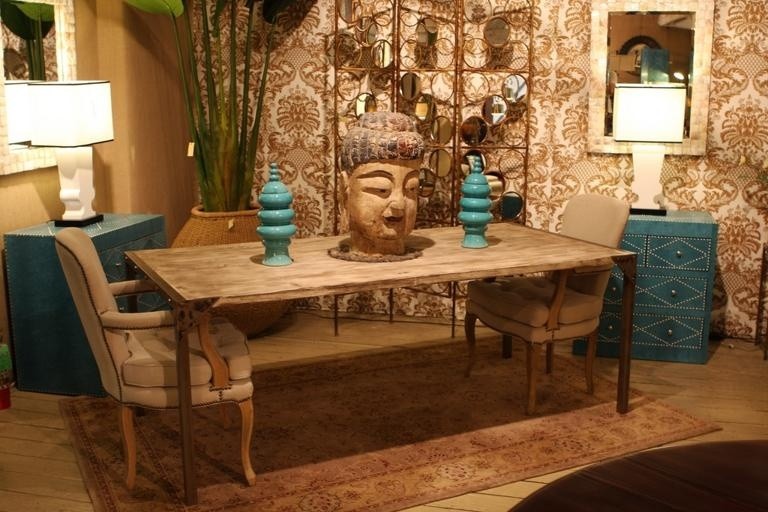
(53, 228), (259, 492)
(461, 193), (631, 415)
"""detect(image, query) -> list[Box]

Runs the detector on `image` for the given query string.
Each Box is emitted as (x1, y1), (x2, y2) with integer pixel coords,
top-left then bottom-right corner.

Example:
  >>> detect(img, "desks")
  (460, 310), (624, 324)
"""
(123, 224), (639, 507)
(508, 440), (766, 510)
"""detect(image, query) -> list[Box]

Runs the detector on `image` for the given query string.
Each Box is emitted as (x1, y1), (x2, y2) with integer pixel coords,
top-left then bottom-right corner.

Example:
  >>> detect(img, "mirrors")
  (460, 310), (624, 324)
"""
(1, 2), (79, 176)
(586, 0), (715, 157)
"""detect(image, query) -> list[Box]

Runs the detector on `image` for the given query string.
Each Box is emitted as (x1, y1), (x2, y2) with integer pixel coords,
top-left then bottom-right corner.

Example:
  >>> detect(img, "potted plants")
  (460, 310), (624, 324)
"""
(127, 0), (291, 338)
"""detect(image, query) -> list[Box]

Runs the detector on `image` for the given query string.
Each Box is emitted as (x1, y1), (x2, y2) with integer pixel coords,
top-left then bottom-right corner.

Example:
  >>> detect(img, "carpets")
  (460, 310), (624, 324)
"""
(58, 333), (727, 510)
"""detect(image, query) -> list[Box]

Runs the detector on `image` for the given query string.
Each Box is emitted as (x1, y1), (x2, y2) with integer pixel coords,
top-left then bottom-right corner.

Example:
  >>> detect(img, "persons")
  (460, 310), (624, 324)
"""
(340, 111), (424, 255)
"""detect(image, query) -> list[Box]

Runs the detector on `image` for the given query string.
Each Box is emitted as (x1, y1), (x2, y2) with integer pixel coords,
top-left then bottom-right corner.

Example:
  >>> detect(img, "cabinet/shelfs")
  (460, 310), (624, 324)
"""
(2, 212), (169, 398)
(570, 209), (719, 365)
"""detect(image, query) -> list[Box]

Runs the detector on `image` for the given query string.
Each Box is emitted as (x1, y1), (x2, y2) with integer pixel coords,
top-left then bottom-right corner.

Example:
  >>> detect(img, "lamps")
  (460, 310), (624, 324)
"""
(26, 76), (114, 227)
(613, 82), (687, 216)
